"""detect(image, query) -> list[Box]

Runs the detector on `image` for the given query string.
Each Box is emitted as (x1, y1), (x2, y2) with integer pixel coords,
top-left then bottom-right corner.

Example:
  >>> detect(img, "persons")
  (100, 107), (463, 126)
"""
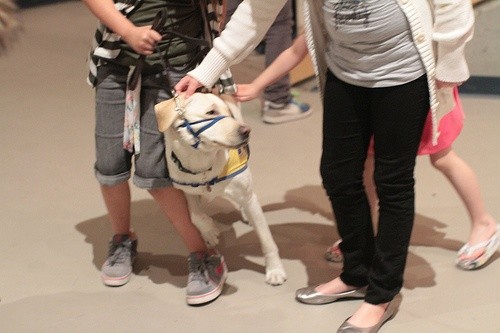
(173, 0), (474, 333)
(83, 0), (228, 306)
(234, 32), (500, 270)
(220, 0), (314, 123)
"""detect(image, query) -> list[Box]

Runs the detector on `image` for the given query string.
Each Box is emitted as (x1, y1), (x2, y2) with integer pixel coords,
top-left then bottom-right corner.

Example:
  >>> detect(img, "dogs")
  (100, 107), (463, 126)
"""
(154, 93), (287, 286)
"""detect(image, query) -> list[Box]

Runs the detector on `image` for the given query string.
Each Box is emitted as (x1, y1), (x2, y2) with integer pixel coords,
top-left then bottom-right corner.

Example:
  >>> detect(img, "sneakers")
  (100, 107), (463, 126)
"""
(263, 97), (312, 123)
(101, 232), (138, 286)
(186, 248), (227, 304)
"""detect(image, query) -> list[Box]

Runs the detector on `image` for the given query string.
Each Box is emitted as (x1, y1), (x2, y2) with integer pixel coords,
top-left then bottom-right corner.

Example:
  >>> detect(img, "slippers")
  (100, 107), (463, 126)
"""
(455, 226), (500, 269)
(326, 239), (344, 262)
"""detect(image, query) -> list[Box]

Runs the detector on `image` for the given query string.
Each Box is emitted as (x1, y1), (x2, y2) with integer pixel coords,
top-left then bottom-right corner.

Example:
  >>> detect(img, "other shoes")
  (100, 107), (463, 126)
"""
(336, 293), (403, 333)
(296, 282), (368, 305)
